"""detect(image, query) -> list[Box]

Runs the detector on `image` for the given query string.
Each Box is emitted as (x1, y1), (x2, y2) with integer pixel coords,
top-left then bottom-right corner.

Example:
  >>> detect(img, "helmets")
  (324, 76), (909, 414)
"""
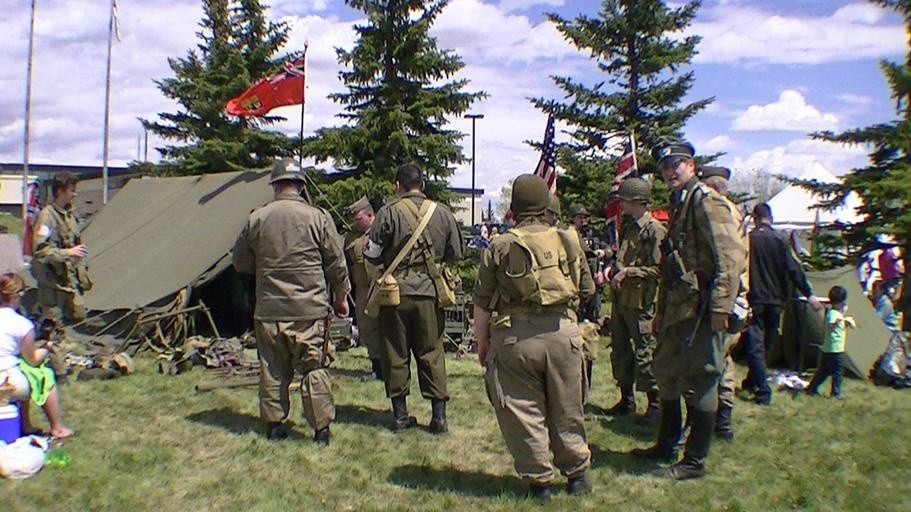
(268, 159), (308, 186)
(617, 179), (654, 205)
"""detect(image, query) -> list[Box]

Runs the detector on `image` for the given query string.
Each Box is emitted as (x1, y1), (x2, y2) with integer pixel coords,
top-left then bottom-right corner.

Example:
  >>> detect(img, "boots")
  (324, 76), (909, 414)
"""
(605, 386), (737, 480)
(389, 395), (450, 434)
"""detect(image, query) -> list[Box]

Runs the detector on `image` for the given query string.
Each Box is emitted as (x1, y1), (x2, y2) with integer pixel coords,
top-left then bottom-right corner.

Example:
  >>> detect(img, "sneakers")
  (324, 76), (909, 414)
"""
(265, 422), (289, 442)
(312, 426), (332, 447)
(526, 478), (592, 504)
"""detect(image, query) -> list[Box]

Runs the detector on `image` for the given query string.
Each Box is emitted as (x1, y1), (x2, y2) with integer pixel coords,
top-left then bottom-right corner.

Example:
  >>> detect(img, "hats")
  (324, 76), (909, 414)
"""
(509, 173), (553, 218)
(344, 195), (372, 215)
(650, 139), (699, 165)
(570, 202), (591, 218)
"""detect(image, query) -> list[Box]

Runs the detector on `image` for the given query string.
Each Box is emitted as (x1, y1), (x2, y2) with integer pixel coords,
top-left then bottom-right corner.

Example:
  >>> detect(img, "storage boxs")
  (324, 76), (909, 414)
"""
(0, 403), (21, 444)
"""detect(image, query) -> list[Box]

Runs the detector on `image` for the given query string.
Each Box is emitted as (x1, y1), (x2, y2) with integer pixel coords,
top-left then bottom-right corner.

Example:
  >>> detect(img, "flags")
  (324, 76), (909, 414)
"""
(223, 55), (304, 117)
(503, 100), (557, 226)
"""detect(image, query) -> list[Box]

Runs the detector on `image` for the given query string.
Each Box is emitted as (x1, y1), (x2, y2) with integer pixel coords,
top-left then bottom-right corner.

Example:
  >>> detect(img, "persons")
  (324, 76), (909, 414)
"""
(32, 172), (89, 387)
(602, 138), (751, 480)
(471, 173), (598, 500)
(343, 196), (384, 381)
(362, 164), (464, 433)
(858, 219), (903, 389)
(735, 204), (856, 403)
(233, 157), (350, 446)
(0, 273), (74, 439)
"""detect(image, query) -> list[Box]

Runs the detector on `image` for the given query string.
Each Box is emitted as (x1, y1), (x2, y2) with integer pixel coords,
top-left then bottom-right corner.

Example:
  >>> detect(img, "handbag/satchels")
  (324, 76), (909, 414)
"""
(433, 266), (459, 306)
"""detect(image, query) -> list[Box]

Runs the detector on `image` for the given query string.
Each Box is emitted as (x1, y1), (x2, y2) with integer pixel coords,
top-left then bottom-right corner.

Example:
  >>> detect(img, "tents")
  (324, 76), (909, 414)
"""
(11, 167), (335, 355)
(746, 159), (869, 236)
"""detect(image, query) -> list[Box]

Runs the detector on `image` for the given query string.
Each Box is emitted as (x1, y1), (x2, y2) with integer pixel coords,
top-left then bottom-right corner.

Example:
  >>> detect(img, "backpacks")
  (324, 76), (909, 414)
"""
(508, 230), (584, 304)
(363, 278), (386, 317)
